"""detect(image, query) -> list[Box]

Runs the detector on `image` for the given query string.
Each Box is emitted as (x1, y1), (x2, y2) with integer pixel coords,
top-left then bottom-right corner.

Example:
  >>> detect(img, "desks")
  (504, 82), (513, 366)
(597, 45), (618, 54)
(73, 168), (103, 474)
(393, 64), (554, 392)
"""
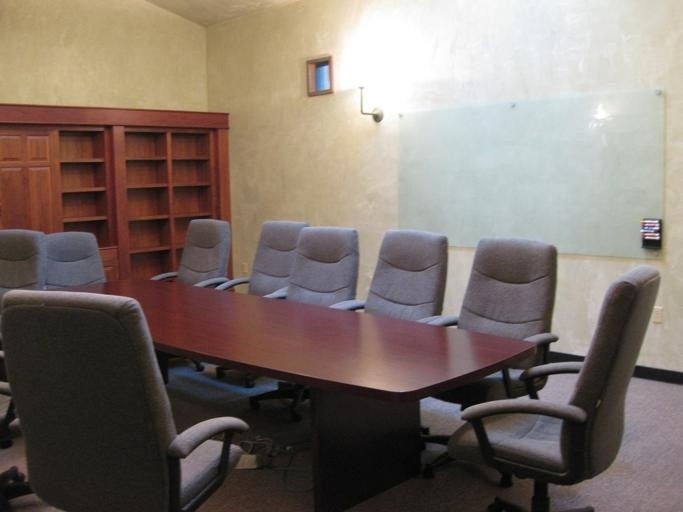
(94, 273), (537, 512)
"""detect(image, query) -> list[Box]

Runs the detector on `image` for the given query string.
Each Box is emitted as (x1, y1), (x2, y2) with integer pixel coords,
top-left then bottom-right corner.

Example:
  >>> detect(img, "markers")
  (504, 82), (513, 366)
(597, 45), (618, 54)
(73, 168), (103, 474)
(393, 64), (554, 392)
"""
(640, 219), (661, 244)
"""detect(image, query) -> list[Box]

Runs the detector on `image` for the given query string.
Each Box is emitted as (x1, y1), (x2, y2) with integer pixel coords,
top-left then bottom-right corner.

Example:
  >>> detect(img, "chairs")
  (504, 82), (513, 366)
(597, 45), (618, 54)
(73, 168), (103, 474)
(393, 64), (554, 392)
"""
(212, 221), (308, 301)
(330, 228), (449, 328)
(1, 227), (107, 292)
(261, 227), (359, 317)
(415, 235), (558, 489)
(446, 267), (662, 512)
(0, 288), (251, 512)
(150, 216), (232, 289)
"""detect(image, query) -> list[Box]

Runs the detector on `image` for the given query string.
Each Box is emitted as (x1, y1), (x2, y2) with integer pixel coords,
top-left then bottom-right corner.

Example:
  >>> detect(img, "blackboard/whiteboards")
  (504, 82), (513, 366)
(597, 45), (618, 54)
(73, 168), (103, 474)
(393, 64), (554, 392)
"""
(395, 88), (666, 259)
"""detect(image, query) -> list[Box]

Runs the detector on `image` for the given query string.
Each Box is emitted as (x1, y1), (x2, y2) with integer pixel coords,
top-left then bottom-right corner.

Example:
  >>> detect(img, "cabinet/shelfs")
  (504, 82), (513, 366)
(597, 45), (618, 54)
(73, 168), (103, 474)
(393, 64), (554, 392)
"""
(0, 102), (234, 289)
